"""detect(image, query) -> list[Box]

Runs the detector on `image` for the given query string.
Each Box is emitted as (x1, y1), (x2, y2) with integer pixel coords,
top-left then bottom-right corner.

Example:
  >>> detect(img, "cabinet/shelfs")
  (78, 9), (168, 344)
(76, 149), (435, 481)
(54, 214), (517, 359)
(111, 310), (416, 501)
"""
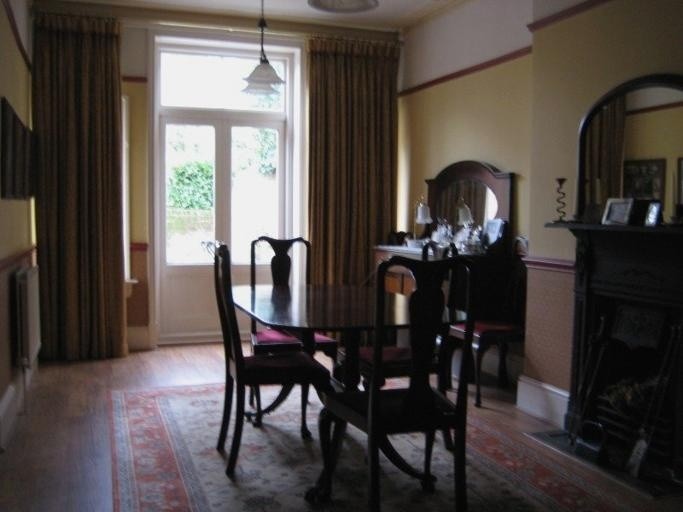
(370, 243), (509, 392)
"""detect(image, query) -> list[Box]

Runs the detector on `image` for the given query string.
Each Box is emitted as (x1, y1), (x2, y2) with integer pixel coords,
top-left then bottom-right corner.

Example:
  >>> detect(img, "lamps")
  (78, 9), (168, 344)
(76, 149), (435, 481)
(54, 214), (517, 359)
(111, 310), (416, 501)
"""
(411, 194), (433, 238)
(451, 193), (473, 232)
(241, 0), (288, 101)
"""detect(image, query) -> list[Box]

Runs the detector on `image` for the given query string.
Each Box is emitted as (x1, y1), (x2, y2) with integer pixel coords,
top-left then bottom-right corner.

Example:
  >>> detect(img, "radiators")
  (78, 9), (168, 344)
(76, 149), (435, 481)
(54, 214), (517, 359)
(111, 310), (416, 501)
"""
(13, 262), (44, 410)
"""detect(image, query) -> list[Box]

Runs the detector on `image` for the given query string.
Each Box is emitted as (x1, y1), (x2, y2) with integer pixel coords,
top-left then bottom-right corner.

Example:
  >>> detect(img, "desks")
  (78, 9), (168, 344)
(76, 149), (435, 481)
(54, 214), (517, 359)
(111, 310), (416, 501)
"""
(228, 284), (467, 440)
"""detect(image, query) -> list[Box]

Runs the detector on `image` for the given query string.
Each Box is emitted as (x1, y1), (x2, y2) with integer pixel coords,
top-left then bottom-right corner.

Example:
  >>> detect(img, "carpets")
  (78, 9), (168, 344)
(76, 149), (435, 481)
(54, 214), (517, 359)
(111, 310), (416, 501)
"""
(106, 359), (671, 511)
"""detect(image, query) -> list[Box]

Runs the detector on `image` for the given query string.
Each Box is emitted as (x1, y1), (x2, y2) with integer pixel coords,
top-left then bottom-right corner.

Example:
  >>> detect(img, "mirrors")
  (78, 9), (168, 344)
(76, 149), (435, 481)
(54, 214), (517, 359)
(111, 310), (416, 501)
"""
(575, 70), (682, 222)
(425, 159), (517, 240)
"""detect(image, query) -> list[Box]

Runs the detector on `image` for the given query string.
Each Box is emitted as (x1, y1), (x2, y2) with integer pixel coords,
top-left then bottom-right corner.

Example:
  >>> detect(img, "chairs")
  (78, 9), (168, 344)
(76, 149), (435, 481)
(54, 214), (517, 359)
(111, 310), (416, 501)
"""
(248, 237), (313, 429)
(217, 243), (332, 477)
(306, 255), (470, 510)
(447, 237), (528, 407)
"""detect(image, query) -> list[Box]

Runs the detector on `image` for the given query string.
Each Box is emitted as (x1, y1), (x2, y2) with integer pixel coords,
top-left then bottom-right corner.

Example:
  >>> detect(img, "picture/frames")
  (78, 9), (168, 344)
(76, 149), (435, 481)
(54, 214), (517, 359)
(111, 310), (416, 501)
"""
(600, 195), (637, 225)
(618, 155), (666, 212)
